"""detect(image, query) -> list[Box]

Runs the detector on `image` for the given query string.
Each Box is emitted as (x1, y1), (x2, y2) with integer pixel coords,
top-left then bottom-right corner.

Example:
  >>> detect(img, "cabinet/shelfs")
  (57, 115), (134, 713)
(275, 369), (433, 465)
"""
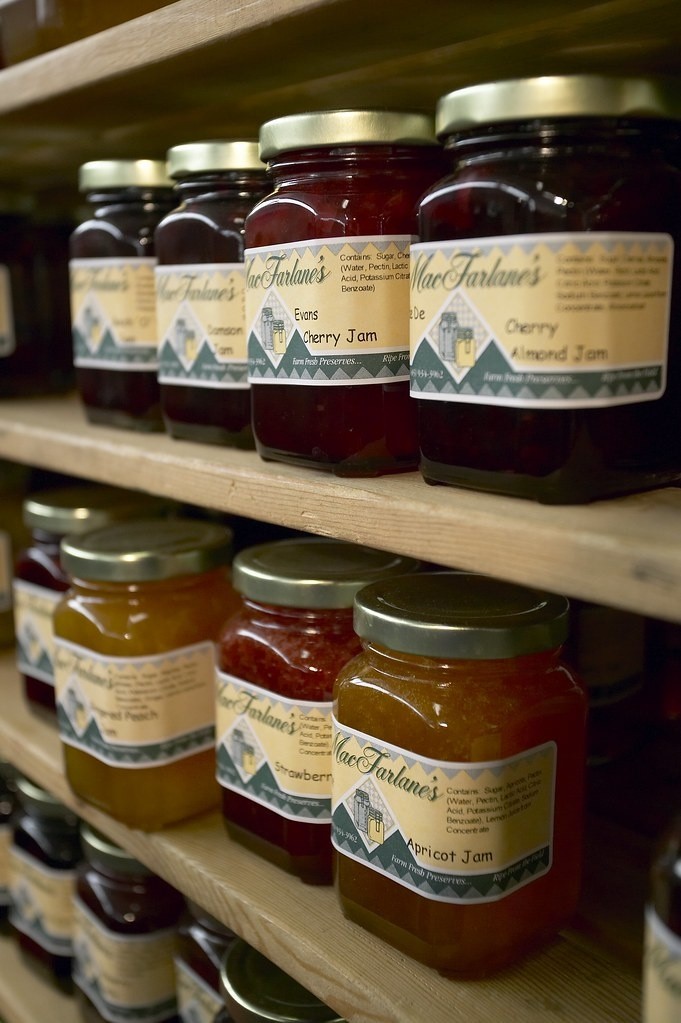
(1, 0), (681, 1022)
